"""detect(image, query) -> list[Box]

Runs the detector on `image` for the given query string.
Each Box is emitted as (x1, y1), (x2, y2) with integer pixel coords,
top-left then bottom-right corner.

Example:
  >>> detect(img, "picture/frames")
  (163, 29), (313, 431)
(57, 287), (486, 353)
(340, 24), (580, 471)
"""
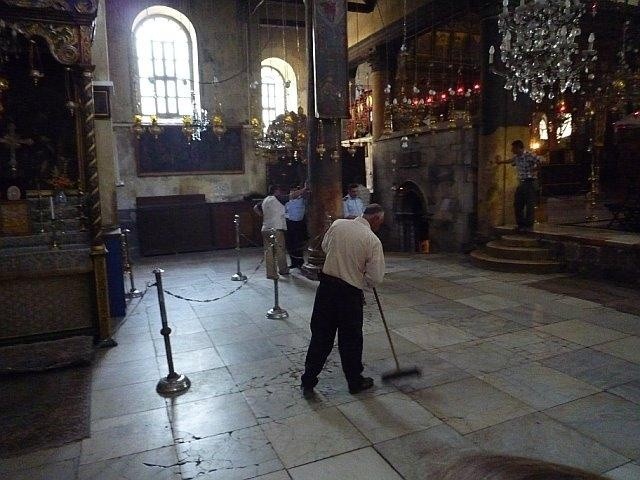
(91, 86), (112, 119)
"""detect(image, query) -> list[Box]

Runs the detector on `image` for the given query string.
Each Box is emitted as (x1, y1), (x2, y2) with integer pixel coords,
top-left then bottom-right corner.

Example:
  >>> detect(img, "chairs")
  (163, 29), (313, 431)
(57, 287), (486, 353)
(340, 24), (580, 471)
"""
(604, 186), (637, 230)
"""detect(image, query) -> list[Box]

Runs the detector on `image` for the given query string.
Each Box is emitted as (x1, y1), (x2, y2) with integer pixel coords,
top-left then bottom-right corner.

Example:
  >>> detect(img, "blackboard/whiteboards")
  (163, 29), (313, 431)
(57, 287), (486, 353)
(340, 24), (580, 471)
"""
(136, 124), (246, 177)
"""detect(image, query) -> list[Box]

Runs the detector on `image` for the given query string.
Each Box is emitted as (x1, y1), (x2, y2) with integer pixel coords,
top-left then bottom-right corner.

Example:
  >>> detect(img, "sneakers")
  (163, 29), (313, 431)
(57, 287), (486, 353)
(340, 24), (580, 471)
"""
(301, 380), (313, 399)
(350, 377), (373, 393)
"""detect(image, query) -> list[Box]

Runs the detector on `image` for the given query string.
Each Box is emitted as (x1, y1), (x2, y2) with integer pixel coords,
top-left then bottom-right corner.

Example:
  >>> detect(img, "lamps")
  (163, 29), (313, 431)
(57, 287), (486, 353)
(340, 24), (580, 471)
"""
(127, 1), (485, 165)
(486, 0), (601, 107)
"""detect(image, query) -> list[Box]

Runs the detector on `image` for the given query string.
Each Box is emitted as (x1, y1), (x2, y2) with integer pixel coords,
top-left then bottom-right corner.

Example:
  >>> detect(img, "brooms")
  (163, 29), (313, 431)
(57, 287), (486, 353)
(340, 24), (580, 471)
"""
(372, 282), (423, 385)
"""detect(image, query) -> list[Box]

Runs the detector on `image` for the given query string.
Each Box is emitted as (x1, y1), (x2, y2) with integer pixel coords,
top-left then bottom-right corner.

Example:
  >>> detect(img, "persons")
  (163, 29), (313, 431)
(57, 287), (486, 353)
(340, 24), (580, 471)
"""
(495, 140), (542, 230)
(342, 180), (366, 220)
(302, 203), (388, 399)
(284, 183), (307, 270)
(252, 176), (315, 277)
